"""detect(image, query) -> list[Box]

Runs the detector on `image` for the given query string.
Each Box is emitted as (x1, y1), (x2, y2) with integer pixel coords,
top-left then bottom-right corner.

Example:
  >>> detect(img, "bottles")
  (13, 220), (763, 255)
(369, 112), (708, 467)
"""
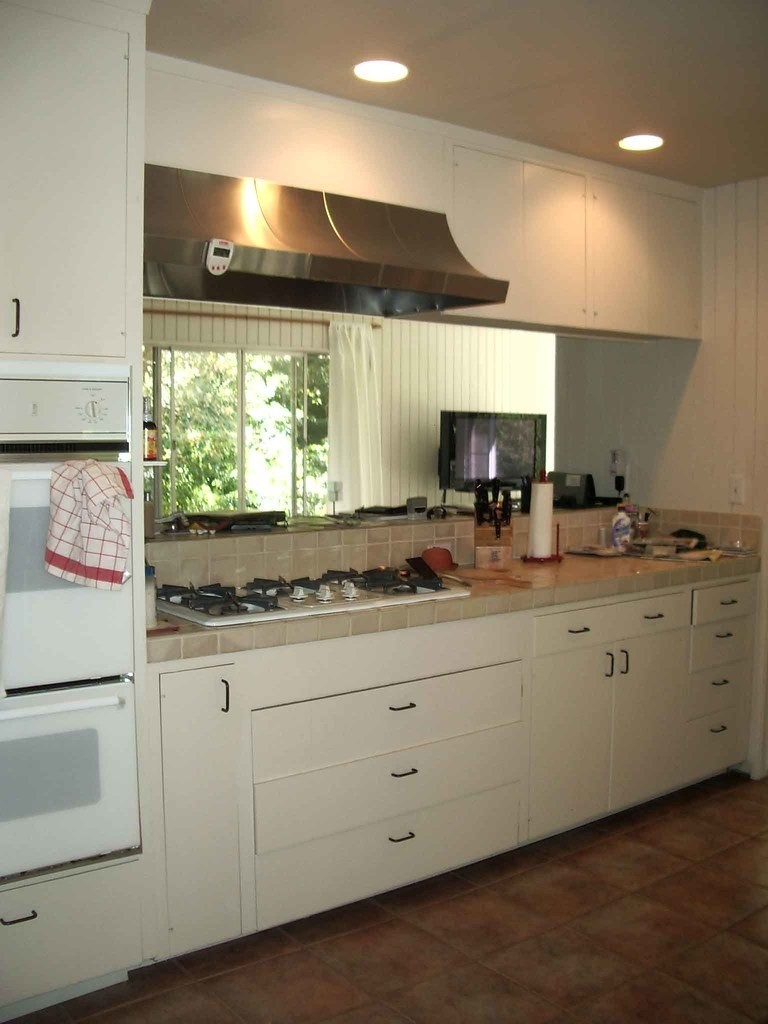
(612, 507), (631, 552)
(144, 397), (158, 460)
(146, 565), (158, 627)
(143, 492), (154, 541)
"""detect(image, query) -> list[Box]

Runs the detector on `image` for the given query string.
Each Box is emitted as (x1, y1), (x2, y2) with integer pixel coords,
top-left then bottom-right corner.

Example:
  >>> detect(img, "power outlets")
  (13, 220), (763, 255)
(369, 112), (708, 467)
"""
(617, 463), (629, 493)
(729, 472), (746, 506)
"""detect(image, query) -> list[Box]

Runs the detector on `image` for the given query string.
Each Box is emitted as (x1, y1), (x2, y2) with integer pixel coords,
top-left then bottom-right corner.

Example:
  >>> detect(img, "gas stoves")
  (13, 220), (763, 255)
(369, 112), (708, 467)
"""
(161, 559), (474, 629)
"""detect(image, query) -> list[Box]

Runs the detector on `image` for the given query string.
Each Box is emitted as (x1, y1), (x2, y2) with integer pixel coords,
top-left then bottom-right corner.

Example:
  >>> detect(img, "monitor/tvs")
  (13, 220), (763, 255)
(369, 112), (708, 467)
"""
(441, 411), (546, 490)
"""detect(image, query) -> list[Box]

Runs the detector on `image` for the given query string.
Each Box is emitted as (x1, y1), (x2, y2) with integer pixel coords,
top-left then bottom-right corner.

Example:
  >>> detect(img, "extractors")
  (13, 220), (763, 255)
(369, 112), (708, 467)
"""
(145, 165), (509, 315)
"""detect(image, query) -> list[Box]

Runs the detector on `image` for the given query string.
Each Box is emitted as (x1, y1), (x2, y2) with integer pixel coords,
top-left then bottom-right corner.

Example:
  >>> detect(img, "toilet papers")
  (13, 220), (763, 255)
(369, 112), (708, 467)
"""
(528, 479), (554, 557)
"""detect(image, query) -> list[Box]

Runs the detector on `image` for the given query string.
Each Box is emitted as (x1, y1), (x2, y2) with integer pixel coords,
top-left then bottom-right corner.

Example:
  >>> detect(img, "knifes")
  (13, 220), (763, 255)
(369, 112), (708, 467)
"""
(477, 480), (513, 527)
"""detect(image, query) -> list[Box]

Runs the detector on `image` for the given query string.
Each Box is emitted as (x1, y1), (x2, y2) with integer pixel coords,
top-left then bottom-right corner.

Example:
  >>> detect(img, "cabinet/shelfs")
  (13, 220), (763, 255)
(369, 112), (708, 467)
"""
(444, 124), (704, 341)
(146, 543), (762, 996)
(0, 0), (153, 1024)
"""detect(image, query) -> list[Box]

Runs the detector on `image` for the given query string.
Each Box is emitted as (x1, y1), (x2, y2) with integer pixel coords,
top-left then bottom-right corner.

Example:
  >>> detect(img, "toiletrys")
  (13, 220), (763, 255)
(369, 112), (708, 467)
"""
(611, 493), (652, 552)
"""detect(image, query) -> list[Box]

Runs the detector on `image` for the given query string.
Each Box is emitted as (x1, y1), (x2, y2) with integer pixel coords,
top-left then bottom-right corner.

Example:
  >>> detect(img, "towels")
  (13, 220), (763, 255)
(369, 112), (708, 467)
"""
(45, 458), (133, 592)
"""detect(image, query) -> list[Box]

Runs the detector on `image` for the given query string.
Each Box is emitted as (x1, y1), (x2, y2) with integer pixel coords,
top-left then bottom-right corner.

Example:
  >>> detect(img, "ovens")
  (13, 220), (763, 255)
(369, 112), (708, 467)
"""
(0, 364), (140, 879)
